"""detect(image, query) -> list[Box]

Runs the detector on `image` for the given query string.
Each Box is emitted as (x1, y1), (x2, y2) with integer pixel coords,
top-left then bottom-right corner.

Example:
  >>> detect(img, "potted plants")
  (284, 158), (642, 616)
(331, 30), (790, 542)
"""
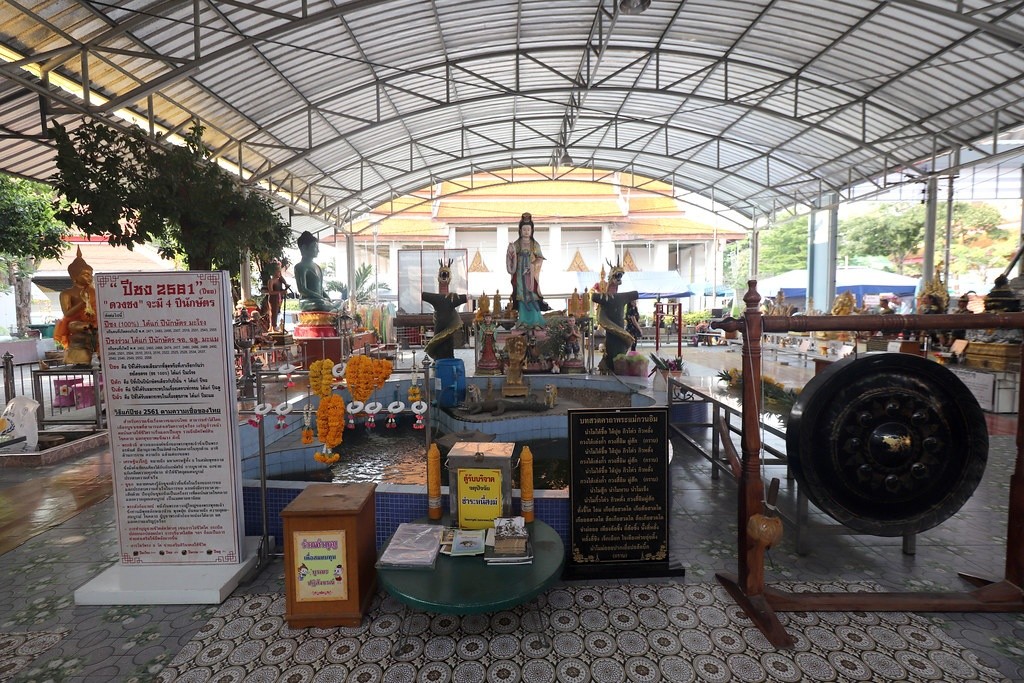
(613, 353), (628, 374)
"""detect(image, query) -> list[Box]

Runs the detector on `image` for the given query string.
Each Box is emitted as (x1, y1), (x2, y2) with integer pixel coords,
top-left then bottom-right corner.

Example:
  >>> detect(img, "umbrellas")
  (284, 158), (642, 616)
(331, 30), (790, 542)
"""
(703, 285), (734, 297)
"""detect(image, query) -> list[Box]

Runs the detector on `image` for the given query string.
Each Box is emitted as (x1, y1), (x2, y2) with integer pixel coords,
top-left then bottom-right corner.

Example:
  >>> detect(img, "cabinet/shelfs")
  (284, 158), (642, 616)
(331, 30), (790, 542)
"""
(721, 331), (856, 369)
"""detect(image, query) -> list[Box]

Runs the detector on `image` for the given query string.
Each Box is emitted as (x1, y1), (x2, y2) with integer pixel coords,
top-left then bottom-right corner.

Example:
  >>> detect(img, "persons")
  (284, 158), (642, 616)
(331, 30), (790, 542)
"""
(922, 294), (940, 313)
(506, 212), (551, 327)
(886, 293), (914, 340)
(949, 292), (973, 340)
(58, 243), (100, 349)
(291, 229), (344, 315)
(624, 300), (643, 351)
(880, 298), (894, 315)
(478, 312), (501, 369)
(572, 288), (582, 316)
(591, 270), (608, 320)
(263, 261), (289, 333)
(581, 287), (591, 315)
(494, 289), (501, 312)
(563, 314), (582, 360)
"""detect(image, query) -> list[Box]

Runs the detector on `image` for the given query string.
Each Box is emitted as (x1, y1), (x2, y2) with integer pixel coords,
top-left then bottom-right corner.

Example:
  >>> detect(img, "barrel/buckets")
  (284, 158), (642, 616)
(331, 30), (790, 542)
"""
(653, 369), (683, 390)
(436, 359), (465, 408)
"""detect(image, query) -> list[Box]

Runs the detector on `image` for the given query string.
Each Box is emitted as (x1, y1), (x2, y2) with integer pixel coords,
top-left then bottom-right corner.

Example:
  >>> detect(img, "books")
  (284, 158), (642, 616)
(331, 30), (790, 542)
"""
(439, 516), (533, 565)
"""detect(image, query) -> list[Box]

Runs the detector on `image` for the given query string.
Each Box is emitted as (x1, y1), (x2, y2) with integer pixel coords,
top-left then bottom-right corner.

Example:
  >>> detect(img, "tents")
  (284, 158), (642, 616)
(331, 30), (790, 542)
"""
(754, 267), (920, 307)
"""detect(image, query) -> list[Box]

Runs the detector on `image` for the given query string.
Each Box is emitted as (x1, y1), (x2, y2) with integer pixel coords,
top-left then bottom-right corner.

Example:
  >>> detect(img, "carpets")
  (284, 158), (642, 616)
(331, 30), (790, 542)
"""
(0, 630), (73, 682)
(145, 582), (1011, 683)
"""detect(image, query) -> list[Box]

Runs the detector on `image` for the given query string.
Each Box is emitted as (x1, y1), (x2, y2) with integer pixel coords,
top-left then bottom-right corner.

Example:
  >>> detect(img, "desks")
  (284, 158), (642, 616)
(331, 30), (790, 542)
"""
(669, 374), (918, 556)
(698, 333), (721, 346)
(375, 508), (565, 613)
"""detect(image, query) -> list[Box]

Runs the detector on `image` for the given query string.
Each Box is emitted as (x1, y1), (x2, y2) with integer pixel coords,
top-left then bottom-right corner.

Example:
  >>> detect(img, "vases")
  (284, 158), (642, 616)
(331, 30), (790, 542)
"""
(766, 397), (778, 404)
(660, 370), (682, 387)
(630, 361), (648, 377)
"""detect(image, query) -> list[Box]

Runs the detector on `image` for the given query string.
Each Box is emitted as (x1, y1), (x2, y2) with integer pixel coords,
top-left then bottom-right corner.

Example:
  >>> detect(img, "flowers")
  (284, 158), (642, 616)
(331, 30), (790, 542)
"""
(695, 323), (709, 334)
(247, 354), (423, 465)
(649, 351), (685, 377)
(626, 350), (649, 363)
(716, 368), (806, 414)
